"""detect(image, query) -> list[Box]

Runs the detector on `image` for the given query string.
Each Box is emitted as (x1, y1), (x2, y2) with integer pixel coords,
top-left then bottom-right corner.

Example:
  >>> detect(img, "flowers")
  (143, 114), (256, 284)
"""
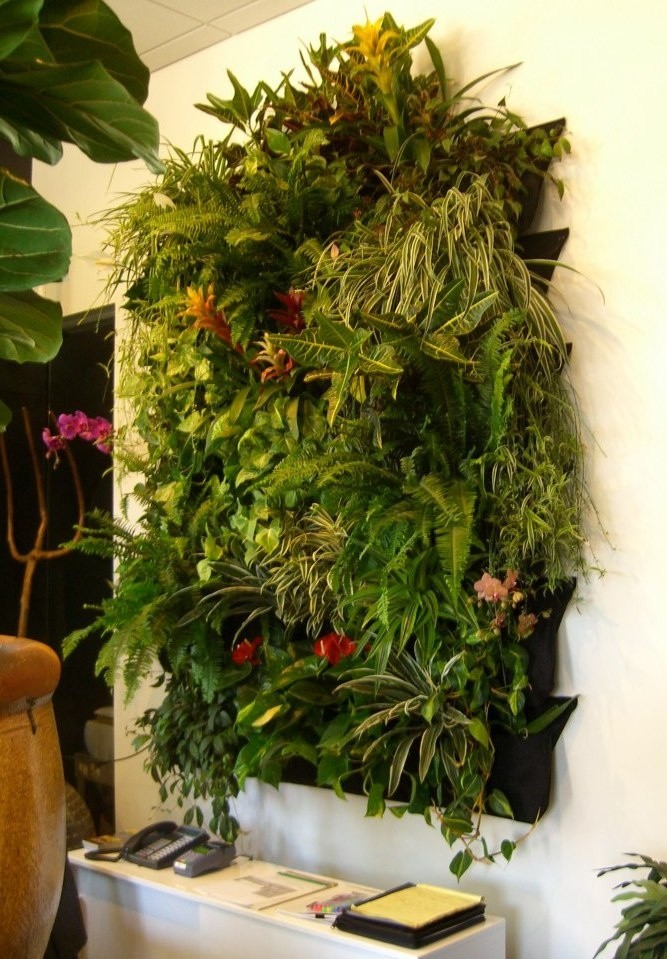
(43, 6), (616, 882)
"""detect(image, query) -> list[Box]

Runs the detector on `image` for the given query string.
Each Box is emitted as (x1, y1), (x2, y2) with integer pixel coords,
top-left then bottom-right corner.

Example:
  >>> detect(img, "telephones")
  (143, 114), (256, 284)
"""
(120, 819), (210, 871)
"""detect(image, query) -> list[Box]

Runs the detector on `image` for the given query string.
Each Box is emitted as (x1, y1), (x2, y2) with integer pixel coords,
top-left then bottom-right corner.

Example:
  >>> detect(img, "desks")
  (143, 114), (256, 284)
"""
(66, 835), (505, 959)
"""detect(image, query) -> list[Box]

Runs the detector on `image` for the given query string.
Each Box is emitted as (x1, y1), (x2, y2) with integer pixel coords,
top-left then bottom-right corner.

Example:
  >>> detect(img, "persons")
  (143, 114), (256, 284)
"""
(44, 779), (98, 959)
